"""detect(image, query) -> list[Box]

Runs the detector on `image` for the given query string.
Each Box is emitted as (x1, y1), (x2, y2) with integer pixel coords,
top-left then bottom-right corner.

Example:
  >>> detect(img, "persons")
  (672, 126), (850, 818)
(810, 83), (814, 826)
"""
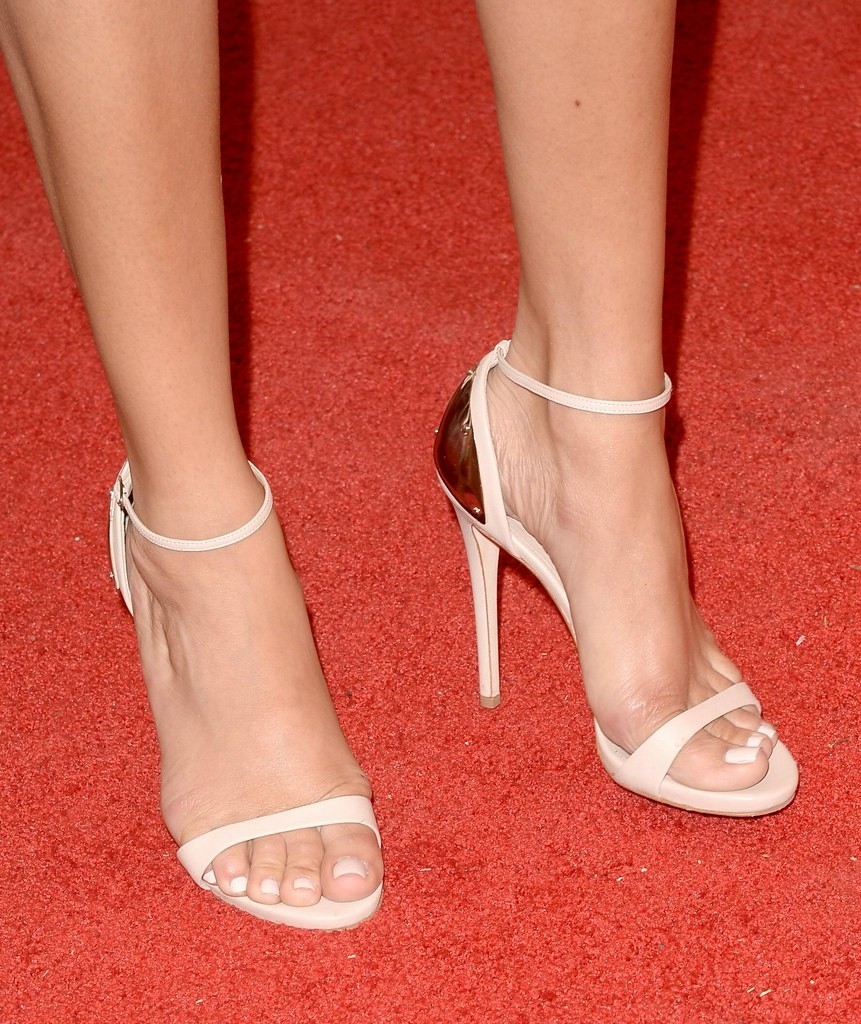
(0, 0), (797, 931)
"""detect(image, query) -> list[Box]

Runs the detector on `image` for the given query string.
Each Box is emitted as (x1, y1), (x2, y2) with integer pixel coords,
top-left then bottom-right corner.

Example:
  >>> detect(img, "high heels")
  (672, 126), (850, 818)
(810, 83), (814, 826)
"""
(436, 334), (800, 817)
(107, 456), (383, 929)
